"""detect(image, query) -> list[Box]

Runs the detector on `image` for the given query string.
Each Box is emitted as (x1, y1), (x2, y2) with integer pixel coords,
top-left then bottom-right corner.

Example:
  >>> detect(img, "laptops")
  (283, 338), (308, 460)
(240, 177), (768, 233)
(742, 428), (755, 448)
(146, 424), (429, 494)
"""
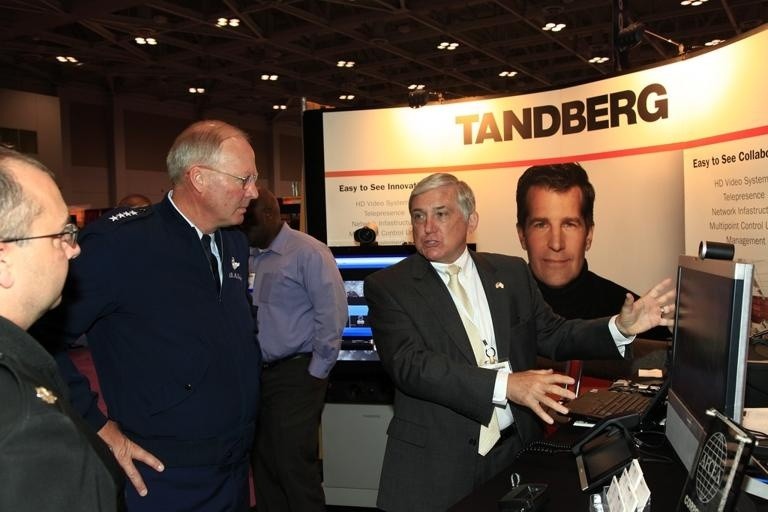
(555, 373), (669, 427)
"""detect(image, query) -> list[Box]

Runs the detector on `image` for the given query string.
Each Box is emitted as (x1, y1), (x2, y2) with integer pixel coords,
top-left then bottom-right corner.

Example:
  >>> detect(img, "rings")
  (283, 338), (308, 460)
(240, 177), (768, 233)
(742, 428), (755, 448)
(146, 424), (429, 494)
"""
(661, 306), (665, 316)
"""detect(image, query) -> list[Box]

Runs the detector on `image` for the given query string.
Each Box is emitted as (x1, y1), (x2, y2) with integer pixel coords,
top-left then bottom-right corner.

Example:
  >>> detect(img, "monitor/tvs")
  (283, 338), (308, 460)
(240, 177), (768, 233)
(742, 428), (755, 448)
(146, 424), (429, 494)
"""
(664, 254), (755, 473)
(673, 407), (758, 512)
(576, 435), (642, 494)
(326, 242), (477, 361)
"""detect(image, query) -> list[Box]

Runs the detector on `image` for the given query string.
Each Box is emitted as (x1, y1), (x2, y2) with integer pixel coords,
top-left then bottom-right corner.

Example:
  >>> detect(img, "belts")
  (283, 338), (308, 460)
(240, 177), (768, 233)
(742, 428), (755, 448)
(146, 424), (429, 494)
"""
(273, 352), (313, 368)
(501, 422), (519, 438)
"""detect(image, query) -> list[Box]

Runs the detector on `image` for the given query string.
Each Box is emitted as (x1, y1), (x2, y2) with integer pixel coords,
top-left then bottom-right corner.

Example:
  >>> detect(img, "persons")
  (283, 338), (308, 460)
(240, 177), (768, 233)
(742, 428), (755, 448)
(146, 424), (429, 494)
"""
(516, 162), (672, 341)
(0, 141), (120, 512)
(363, 173), (677, 512)
(242, 185), (349, 512)
(27, 119), (261, 512)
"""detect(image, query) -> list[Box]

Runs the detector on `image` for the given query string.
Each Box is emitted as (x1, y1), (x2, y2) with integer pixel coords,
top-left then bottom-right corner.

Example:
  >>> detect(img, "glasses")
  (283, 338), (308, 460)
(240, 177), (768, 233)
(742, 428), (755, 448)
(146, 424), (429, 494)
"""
(0, 223), (79, 245)
(187, 166), (258, 190)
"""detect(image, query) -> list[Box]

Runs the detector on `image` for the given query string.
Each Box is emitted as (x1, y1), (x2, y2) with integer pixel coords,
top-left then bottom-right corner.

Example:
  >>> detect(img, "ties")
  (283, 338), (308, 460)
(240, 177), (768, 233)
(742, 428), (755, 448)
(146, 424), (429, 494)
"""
(446, 266), (501, 459)
(200, 234), (221, 298)
